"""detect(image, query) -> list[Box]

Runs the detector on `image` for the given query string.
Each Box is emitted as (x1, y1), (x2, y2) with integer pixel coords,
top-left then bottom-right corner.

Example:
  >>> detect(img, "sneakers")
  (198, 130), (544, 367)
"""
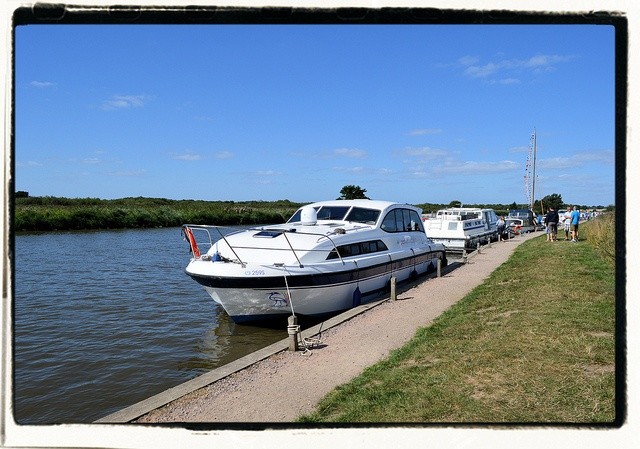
(569, 239), (576, 243)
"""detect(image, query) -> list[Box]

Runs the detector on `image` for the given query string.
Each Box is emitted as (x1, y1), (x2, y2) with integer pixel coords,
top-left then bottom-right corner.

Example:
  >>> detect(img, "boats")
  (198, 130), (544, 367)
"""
(422, 207), (500, 254)
(502, 209), (541, 234)
(181, 198), (446, 327)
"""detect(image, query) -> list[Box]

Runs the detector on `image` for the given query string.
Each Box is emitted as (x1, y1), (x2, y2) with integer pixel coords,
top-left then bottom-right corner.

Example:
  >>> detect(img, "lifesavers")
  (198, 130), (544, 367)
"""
(514, 226), (522, 235)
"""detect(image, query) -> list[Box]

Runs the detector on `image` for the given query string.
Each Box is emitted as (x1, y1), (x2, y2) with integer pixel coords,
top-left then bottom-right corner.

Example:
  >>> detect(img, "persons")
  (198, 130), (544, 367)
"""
(580, 209), (603, 220)
(563, 206), (573, 240)
(496, 216), (506, 242)
(547, 207), (559, 241)
(570, 206), (580, 242)
(545, 209), (554, 241)
